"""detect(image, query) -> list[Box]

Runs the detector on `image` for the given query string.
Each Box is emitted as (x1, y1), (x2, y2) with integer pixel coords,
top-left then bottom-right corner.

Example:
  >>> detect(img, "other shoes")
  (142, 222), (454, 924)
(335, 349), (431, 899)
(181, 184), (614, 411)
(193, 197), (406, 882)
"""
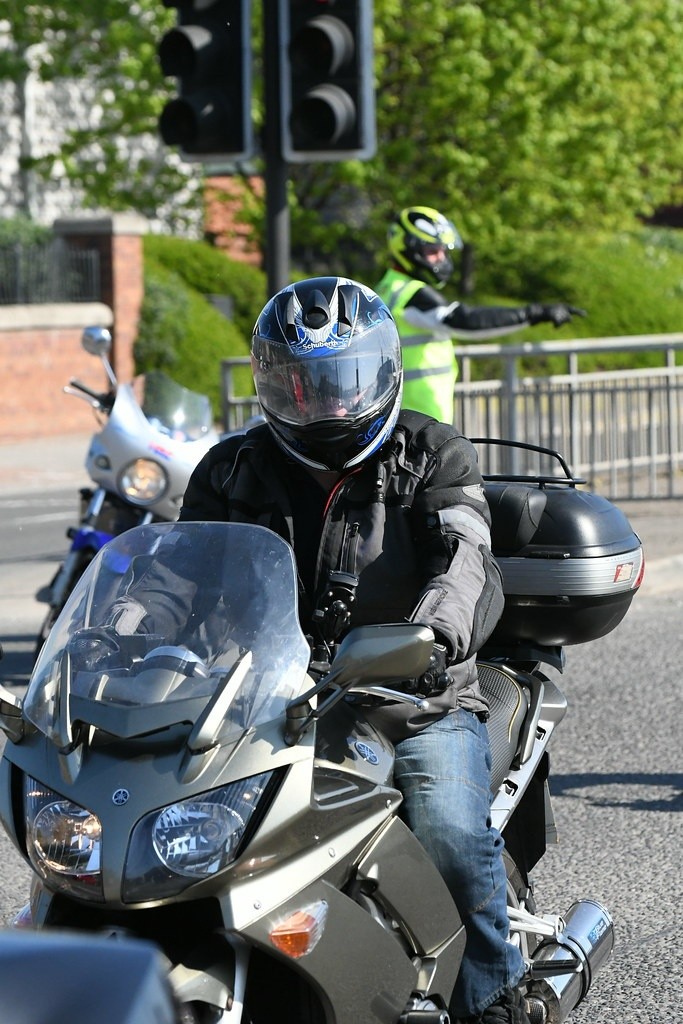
(465, 987), (532, 1024)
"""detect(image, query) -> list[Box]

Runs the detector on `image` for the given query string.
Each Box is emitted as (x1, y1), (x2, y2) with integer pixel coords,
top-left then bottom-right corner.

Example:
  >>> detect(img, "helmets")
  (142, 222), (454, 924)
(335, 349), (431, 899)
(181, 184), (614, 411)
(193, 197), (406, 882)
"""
(250, 274), (404, 471)
(386, 204), (463, 290)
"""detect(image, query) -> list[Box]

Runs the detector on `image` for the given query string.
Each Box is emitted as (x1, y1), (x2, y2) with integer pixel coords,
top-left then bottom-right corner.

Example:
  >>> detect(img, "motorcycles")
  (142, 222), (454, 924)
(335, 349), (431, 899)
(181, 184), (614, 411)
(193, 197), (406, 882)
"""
(0, 435), (639, 1024)
(36, 323), (286, 665)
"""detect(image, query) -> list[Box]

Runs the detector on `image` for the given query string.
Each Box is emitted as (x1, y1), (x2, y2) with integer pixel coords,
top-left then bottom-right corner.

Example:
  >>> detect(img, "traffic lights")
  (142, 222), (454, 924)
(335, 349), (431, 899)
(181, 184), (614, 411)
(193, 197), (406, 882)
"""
(157, 0), (254, 164)
(265, 0), (378, 167)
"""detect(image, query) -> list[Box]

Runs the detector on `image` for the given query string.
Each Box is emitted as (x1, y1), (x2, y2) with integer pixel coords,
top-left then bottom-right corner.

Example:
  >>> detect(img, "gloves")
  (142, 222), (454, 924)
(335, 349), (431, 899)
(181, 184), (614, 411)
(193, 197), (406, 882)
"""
(526, 302), (587, 328)
(386, 638), (448, 696)
(67, 624), (133, 673)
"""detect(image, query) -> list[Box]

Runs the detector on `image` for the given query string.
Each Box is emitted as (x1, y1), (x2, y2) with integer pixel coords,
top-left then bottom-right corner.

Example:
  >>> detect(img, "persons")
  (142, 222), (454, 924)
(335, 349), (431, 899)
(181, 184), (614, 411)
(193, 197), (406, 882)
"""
(63, 275), (536, 1023)
(370, 205), (589, 426)
